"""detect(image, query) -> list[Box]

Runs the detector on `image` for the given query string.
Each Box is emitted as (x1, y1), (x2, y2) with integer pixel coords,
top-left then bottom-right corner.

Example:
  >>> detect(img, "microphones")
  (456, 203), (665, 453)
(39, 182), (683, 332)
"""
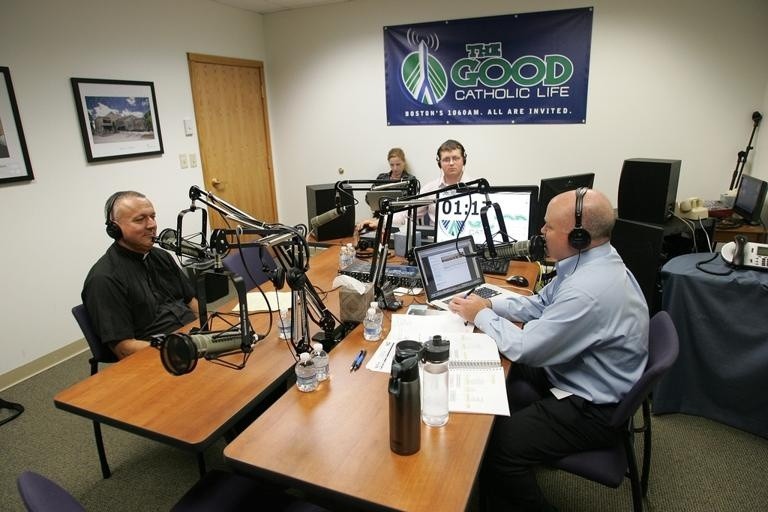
(161, 329), (264, 376)
(478, 236), (546, 260)
(159, 228), (215, 260)
(310, 206), (351, 228)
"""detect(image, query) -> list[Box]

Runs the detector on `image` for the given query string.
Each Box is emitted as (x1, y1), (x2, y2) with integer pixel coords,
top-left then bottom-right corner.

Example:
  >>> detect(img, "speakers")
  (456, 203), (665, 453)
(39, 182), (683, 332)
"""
(306, 183), (355, 241)
(618, 158), (682, 222)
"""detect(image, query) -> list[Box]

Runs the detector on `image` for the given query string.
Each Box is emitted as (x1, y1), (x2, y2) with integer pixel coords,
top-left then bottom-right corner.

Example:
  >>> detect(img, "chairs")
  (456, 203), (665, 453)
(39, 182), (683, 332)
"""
(71, 303), (128, 479)
(221, 240), (276, 291)
(507, 308), (683, 510)
(16, 469), (327, 512)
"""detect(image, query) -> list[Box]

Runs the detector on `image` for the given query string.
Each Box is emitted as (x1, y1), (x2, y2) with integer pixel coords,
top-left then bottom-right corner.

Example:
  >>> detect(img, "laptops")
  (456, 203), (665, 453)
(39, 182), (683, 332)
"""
(413, 235), (523, 321)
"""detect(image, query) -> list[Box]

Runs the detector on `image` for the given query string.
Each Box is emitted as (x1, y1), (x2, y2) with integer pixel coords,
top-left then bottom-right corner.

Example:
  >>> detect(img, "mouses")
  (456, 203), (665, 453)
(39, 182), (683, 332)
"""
(506, 275), (528, 287)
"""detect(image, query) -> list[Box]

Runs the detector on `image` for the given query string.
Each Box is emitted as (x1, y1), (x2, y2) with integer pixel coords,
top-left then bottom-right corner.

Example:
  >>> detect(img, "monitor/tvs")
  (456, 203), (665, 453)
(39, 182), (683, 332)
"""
(435, 185), (538, 264)
(732, 174), (768, 225)
(539, 172), (595, 238)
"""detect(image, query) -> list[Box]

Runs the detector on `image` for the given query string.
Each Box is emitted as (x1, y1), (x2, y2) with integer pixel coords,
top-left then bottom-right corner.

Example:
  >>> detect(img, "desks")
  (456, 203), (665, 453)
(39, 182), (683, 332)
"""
(302, 219), (360, 248)
(661, 216), (714, 261)
(221, 245), (540, 512)
(714, 217), (766, 246)
(649, 252), (767, 439)
(49, 246), (416, 490)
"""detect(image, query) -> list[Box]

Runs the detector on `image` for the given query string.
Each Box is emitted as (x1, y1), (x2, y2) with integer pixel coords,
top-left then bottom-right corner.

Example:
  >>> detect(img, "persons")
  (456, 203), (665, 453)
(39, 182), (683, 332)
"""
(364, 149), (419, 218)
(356, 139), (479, 250)
(448, 187), (650, 512)
(80, 190), (209, 362)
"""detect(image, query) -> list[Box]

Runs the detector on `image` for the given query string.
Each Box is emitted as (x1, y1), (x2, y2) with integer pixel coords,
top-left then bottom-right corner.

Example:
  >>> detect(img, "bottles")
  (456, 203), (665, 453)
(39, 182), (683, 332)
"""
(339, 243), (355, 269)
(421, 335), (450, 428)
(310, 344), (329, 381)
(363, 301), (384, 341)
(388, 357), (421, 456)
(277, 307), (292, 339)
(294, 351), (318, 392)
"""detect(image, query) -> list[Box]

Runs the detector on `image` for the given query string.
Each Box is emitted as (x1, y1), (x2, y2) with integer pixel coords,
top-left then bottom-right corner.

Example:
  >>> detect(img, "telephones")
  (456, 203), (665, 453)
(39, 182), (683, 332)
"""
(721, 235), (768, 272)
(674, 198), (708, 220)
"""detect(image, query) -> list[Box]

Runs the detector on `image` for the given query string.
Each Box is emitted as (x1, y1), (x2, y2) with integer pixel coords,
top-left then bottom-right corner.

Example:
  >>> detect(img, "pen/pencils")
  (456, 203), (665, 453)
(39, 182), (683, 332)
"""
(463, 288), (475, 298)
(349, 350), (366, 372)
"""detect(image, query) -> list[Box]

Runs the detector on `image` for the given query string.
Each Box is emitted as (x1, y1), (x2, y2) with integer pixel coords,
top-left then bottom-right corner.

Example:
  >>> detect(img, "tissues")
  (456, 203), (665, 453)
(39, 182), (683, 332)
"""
(394, 225), (422, 257)
(332, 274), (375, 322)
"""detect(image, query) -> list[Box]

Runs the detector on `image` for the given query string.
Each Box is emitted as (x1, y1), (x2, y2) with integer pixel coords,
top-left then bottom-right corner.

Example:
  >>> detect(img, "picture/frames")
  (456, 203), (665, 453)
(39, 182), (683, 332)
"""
(69, 76), (165, 162)
(0, 66), (36, 183)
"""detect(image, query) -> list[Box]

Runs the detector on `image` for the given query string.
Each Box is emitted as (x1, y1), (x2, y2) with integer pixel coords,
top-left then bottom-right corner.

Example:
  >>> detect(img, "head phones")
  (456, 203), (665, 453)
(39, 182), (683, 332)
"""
(436, 139), (467, 166)
(568, 186), (591, 248)
(105, 192), (122, 240)
(260, 225), (308, 290)
